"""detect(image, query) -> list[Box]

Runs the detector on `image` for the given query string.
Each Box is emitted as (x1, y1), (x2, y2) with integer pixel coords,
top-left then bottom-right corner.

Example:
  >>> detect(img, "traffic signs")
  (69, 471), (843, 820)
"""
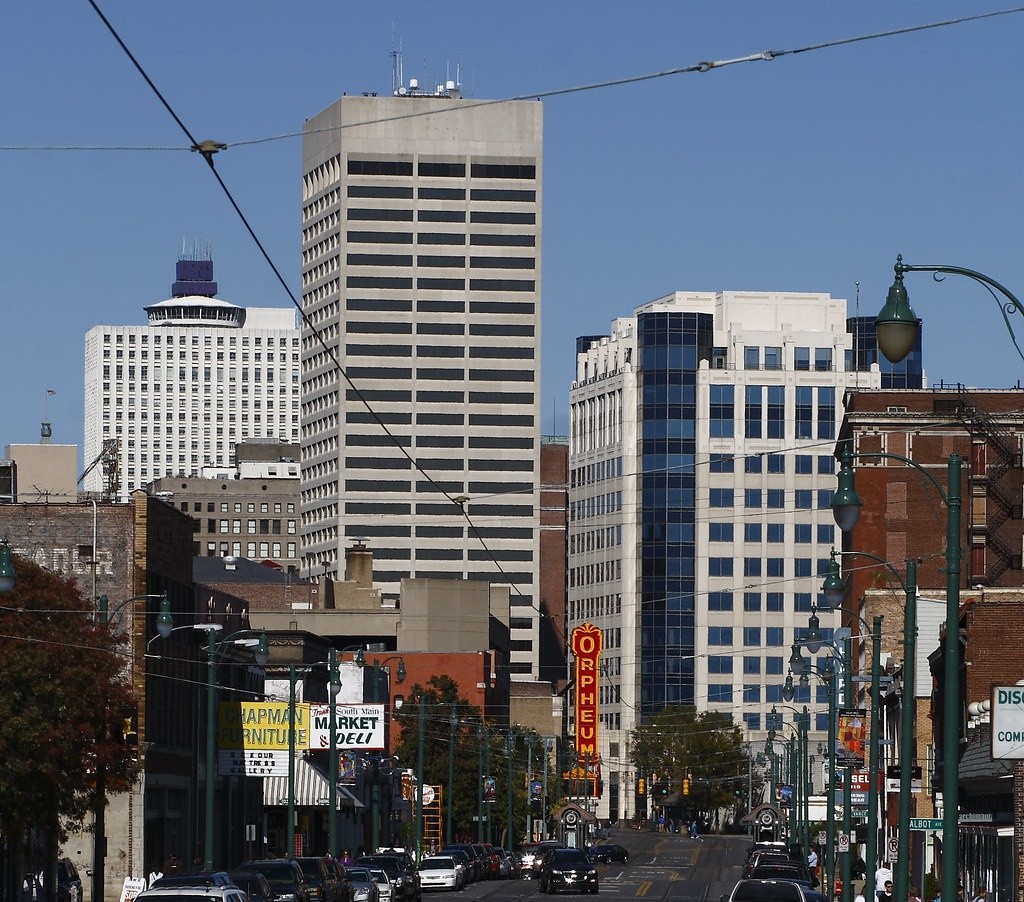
(851, 811), (869, 817)
(908, 816), (944, 830)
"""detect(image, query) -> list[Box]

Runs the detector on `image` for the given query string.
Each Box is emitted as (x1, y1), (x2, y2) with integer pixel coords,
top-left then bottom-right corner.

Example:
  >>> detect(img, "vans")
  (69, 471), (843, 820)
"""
(26, 859), (82, 902)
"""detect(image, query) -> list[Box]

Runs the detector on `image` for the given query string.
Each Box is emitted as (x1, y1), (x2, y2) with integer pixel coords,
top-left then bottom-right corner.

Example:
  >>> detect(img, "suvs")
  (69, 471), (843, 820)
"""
(538, 848), (598, 895)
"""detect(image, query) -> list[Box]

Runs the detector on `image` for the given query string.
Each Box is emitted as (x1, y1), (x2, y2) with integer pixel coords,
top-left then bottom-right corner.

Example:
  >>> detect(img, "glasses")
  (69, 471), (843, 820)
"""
(886, 886), (892, 889)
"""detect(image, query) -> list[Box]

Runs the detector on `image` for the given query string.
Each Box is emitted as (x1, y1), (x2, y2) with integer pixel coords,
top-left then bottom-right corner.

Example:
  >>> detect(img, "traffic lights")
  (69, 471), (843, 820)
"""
(734, 780), (740, 795)
(662, 784), (666, 794)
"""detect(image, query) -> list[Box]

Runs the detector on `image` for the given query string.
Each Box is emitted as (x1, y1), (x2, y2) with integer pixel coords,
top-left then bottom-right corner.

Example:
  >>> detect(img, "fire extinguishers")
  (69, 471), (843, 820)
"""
(836, 880), (841, 895)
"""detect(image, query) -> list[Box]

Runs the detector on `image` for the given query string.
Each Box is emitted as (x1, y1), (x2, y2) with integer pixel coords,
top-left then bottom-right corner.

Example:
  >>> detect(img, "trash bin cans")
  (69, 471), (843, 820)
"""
(838, 883), (856, 902)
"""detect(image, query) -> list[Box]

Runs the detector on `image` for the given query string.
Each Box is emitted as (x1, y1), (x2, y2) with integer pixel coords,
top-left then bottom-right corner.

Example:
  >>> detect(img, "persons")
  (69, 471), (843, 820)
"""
(148, 851), (205, 890)
(658, 815), (700, 839)
(808, 845), (820, 887)
(854, 862), (987, 902)
(339, 848), (353, 866)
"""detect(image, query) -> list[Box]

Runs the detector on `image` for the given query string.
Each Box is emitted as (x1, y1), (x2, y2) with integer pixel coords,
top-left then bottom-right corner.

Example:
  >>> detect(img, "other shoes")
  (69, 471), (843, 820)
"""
(691, 837), (694, 838)
(696, 836), (699, 838)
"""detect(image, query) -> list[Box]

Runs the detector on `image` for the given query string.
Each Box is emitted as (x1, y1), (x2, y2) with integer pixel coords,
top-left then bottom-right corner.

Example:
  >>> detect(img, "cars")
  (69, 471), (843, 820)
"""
(130, 852), (420, 902)
(418, 857), (462, 888)
(720, 840), (825, 902)
(432, 842), (565, 883)
(588, 844), (629, 864)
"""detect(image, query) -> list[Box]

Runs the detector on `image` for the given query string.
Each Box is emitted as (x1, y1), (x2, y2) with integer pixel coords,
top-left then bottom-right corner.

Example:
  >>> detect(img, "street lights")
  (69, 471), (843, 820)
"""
(820, 549), (916, 901)
(91, 593), (173, 901)
(806, 604), (881, 902)
(286, 660), (343, 859)
(766, 616), (852, 902)
(827, 445), (959, 902)
(205, 627), (271, 873)
(372, 656), (606, 858)
